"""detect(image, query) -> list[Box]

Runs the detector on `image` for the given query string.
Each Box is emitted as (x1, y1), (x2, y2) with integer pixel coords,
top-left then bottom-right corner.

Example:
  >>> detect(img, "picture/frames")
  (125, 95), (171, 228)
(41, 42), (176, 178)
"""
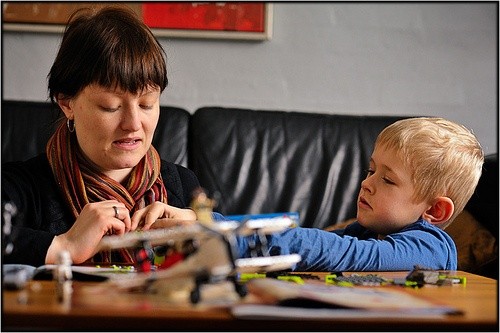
(1, 1), (273, 41)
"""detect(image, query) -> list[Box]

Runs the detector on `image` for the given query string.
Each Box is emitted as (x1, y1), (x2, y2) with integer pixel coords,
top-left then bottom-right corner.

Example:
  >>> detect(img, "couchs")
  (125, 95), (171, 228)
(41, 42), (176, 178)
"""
(1, 99), (482, 269)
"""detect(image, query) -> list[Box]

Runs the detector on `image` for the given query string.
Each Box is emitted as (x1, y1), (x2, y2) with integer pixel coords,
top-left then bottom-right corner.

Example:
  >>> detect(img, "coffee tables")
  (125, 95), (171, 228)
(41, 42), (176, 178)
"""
(2, 266), (498, 331)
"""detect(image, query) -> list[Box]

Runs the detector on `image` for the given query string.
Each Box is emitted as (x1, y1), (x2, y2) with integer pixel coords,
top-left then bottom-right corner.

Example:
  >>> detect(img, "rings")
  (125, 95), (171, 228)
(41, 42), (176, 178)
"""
(113, 206), (120, 218)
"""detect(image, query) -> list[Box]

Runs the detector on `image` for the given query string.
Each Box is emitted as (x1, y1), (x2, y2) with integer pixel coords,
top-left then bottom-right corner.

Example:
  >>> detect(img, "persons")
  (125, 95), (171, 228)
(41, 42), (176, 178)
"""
(205, 115), (485, 274)
(1, 5), (212, 271)
